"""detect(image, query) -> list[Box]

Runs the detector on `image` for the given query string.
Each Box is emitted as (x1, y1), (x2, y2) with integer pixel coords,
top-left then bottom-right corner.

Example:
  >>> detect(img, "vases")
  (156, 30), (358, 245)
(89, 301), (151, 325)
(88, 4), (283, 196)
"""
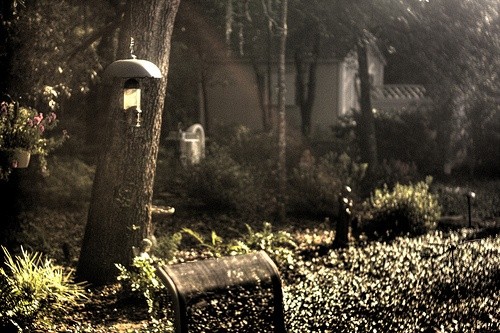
(11, 150), (30, 168)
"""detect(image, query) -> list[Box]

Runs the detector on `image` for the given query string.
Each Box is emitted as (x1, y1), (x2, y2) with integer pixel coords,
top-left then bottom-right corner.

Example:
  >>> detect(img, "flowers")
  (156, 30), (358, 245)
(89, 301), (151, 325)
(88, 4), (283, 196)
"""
(0, 100), (69, 179)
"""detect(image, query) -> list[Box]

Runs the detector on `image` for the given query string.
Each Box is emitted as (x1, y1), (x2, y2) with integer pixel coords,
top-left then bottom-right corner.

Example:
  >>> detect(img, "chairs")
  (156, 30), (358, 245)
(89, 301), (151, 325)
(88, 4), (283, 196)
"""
(157, 250), (287, 333)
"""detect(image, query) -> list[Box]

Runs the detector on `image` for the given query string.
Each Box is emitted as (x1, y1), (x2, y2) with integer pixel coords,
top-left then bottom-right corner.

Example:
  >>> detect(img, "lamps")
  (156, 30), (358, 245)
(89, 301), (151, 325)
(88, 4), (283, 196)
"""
(104, 0), (163, 130)
